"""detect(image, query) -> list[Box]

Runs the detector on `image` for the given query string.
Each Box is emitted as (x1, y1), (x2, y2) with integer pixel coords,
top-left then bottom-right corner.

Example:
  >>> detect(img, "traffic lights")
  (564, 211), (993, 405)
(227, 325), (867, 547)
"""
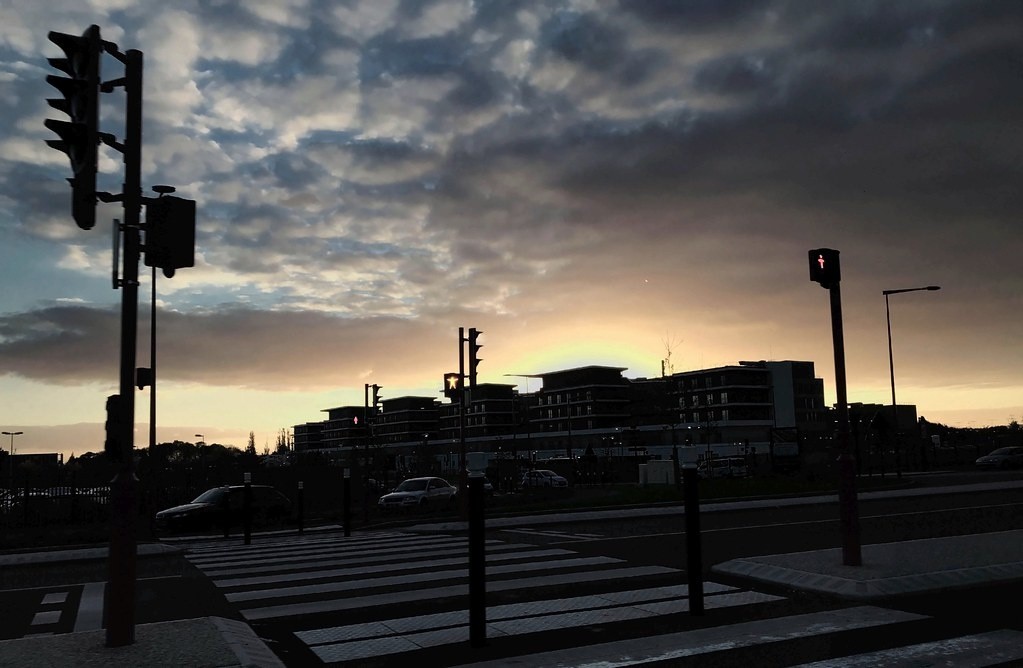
(352, 415), (363, 428)
(45, 22), (105, 231)
(372, 384), (381, 418)
(469, 327), (483, 400)
(810, 248), (831, 283)
(444, 373), (462, 399)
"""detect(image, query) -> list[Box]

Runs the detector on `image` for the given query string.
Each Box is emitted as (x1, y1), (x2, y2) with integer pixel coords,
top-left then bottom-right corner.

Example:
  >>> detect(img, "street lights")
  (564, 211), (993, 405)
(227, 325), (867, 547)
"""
(146, 183), (177, 458)
(614, 442), (622, 456)
(603, 436), (614, 455)
(883, 286), (942, 479)
(1, 432), (23, 488)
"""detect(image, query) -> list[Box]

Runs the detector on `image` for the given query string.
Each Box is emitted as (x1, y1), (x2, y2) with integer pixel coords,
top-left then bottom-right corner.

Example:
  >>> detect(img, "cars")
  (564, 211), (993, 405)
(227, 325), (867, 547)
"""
(378, 476), (459, 511)
(155, 485), (292, 537)
(521, 470), (569, 489)
(975, 446), (1023, 471)
(0, 485), (110, 514)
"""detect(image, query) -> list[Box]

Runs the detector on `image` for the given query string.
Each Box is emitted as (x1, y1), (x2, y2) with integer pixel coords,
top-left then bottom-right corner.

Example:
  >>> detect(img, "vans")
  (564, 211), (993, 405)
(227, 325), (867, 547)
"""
(697, 458), (746, 481)
(465, 473), (494, 498)
